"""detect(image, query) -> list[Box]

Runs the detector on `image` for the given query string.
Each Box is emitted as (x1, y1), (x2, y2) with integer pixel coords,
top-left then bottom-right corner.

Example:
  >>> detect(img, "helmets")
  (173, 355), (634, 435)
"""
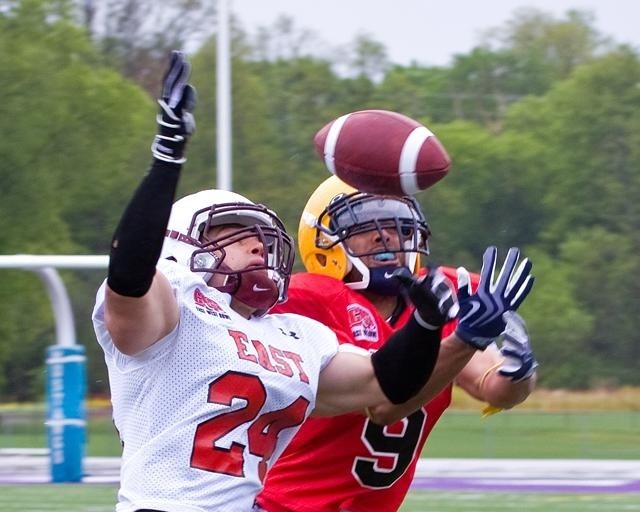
(156, 187), (295, 325)
(297, 171), (433, 289)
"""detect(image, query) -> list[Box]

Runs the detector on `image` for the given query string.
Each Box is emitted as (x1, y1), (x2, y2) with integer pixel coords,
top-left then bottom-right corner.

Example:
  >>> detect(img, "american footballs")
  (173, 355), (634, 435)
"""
(314, 110), (452, 196)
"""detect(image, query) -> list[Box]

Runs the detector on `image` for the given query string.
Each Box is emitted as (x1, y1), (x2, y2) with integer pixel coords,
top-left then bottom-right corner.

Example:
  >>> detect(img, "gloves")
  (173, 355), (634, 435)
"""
(395, 269), (460, 331)
(499, 310), (539, 383)
(150, 48), (198, 163)
(456, 247), (533, 349)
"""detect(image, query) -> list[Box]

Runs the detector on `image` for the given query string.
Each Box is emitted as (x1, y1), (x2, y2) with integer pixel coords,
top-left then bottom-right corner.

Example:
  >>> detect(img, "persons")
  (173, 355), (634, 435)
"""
(254, 172), (538, 512)
(91, 49), (460, 512)
